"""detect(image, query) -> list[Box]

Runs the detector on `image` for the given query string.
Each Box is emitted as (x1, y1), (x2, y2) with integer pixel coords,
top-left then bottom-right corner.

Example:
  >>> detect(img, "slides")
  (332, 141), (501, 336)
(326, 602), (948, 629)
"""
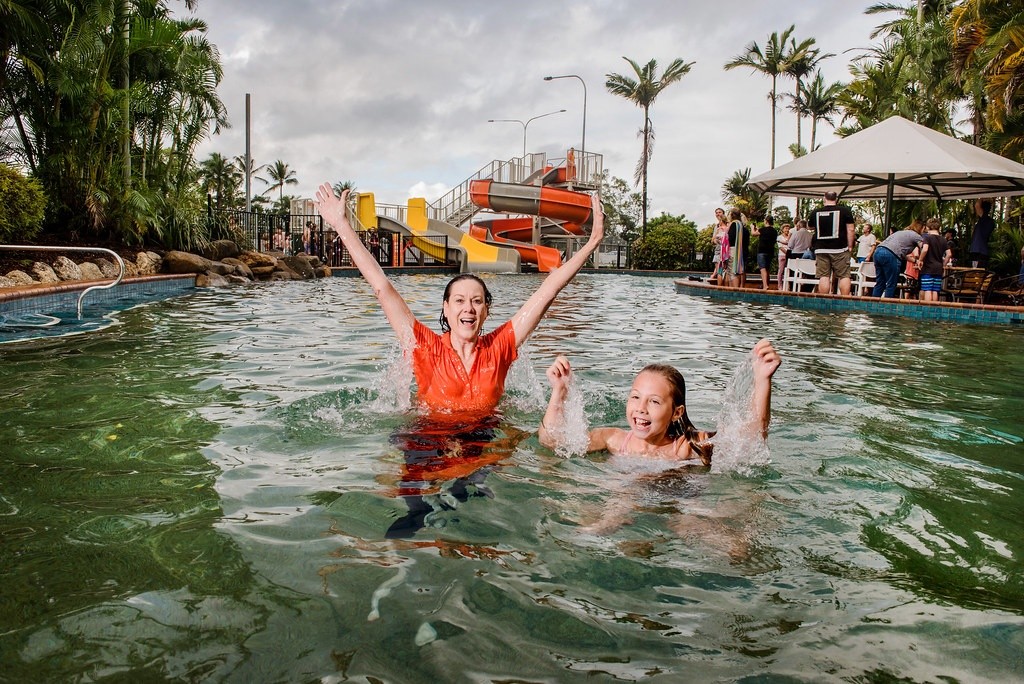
(468, 168), (607, 273)
(375, 213), (521, 274)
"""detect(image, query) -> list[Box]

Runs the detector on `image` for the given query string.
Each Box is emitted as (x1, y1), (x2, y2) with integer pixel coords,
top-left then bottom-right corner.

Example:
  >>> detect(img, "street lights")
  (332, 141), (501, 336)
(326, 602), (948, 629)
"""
(544, 75), (586, 183)
(488, 110), (567, 181)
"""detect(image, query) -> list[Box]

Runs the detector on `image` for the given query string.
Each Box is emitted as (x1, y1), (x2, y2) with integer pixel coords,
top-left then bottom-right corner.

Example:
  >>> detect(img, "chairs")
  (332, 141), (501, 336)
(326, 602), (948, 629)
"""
(783, 259), (995, 304)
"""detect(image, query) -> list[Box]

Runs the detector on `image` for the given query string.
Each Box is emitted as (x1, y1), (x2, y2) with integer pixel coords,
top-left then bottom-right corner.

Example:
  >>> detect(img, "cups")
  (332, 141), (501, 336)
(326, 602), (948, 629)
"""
(973, 261), (977, 268)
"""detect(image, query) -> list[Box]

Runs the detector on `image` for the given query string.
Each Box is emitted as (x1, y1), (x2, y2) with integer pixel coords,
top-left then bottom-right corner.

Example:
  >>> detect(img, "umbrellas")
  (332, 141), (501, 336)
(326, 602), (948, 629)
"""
(742, 116), (1024, 236)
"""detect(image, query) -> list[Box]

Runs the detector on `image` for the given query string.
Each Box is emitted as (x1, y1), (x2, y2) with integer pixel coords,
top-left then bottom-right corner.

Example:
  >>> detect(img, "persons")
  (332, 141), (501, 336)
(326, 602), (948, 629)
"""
(368, 226), (379, 256)
(777, 218), (813, 290)
(855, 224), (876, 293)
(809, 192), (855, 296)
(263, 222), (345, 266)
(711, 207), (749, 287)
(1019, 247), (1024, 284)
(314, 182), (604, 410)
(537, 339), (782, 459)
(751, 216), (777, 290)
(873, 218), (962, 301)
(971, 198), (995, 267)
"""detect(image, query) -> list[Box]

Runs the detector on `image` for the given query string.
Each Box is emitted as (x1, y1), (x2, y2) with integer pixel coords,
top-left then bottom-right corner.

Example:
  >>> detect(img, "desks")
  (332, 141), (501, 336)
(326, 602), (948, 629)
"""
(942, 267), (985, 272)
